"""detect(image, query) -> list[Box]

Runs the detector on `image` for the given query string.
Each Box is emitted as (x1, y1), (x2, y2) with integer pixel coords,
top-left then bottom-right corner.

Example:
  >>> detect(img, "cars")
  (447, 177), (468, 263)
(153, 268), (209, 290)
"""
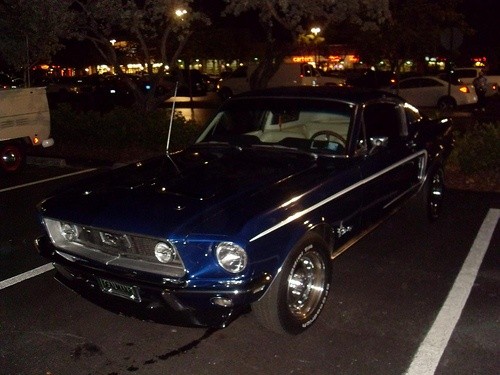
(390, 66), (500, 110)
(35, 91), (453, 338)
(2, 66), (213, 94)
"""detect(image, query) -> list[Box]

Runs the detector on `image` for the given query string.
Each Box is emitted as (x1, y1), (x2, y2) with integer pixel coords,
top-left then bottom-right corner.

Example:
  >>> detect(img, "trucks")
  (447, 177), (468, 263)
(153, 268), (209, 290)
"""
(271, 61), (348, 89)
